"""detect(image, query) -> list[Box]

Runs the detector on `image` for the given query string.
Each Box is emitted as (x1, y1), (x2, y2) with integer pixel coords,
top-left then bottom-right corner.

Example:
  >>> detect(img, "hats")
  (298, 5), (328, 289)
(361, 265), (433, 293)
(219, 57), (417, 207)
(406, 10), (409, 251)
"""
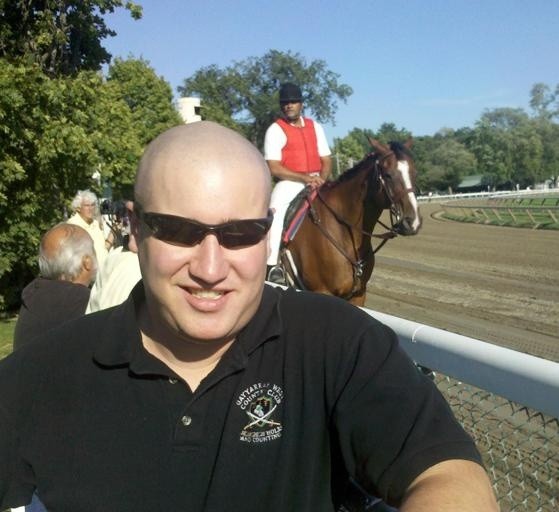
(280, 83), (302, 102)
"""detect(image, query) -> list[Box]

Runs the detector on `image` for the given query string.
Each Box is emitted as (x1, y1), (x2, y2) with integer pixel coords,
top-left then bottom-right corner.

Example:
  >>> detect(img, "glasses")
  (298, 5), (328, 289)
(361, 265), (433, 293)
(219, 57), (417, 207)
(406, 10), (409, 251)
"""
(133, 202), (274, 249)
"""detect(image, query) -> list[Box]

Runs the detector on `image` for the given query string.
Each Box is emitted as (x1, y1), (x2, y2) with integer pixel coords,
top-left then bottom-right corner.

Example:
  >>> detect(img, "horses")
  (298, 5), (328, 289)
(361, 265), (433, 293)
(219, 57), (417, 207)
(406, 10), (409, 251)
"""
(280, 134), (424, 308)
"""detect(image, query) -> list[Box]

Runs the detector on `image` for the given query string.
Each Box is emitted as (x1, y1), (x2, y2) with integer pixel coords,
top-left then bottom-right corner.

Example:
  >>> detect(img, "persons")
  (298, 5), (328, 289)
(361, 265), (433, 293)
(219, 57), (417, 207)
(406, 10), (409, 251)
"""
(14, 224), (97, 510)
(0, 122), (500, 511)
(264, 83), (332, 284)
(68, 190), (118, 257)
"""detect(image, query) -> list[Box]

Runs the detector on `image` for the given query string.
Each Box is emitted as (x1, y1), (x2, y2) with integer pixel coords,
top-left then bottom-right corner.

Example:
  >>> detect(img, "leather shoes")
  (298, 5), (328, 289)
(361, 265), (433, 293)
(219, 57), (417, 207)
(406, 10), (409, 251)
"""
(267, 266), (286, 285)
